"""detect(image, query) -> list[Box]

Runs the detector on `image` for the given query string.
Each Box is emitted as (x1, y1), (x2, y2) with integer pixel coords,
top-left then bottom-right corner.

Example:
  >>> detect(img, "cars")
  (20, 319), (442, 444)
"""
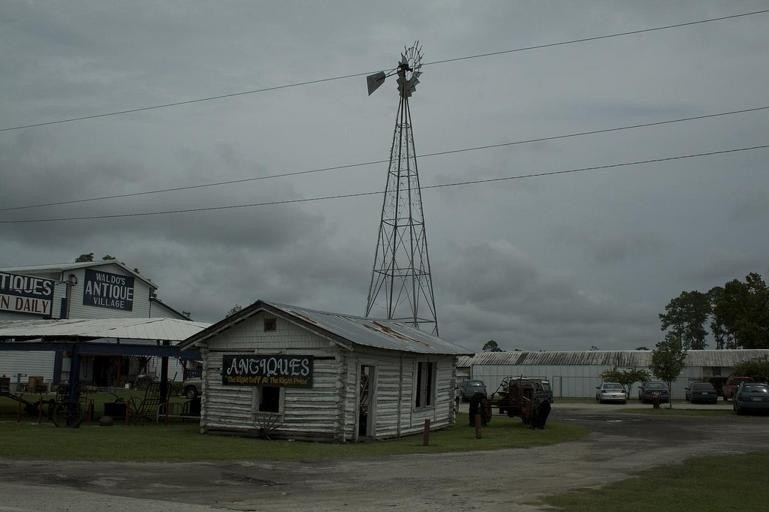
(722, 376), (755, 401)
(119, 373), (160, 391)
(595, 382), (626, 403)
(461, 380), (486, 402)
(181, 378), (202, 399)
(684, 381), (717, 404)
(638, 381), (669, 403)
(733, 381), (769, 415)
(491, 377), (553, 406)
